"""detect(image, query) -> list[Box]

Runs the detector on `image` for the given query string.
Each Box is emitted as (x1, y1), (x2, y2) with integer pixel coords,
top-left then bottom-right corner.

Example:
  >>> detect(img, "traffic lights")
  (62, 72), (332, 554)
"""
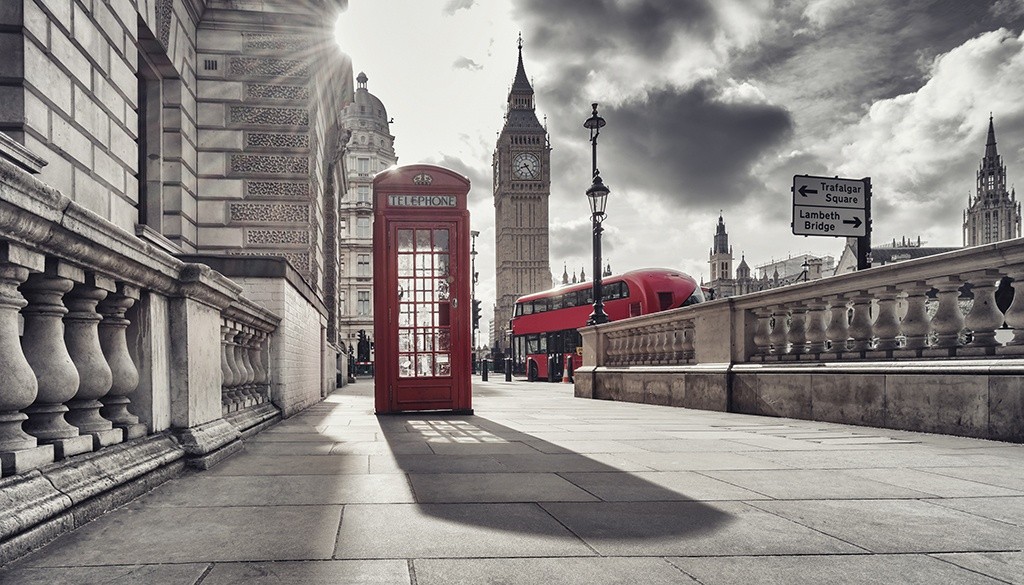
(473, 300), (482, 321)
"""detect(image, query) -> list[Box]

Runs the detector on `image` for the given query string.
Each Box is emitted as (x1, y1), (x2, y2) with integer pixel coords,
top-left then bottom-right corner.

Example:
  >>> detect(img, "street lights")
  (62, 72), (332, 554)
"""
(865, 250), (873, 318)
(585, 169), (611, 326)
(582, 102), (607, 303)
(800, 257), (809, 283)
(470, 231), (481, 374)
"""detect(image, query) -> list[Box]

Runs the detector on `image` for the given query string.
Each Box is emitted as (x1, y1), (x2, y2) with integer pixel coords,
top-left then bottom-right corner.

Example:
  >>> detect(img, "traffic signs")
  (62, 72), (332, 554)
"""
(791, 174), (866, 209)
(792, 205), (866, 249)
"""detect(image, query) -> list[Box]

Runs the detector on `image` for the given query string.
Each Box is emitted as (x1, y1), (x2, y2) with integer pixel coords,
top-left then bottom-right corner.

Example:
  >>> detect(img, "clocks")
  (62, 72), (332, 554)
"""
(510, 150), (543, 181)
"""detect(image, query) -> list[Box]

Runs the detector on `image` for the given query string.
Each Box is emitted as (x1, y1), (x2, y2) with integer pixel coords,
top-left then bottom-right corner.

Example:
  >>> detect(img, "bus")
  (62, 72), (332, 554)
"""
(504, 267), (706, 382)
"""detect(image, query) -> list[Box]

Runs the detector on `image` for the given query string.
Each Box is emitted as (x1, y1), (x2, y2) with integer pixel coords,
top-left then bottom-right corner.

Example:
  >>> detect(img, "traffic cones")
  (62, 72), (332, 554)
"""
(560, 363), (572, 384)
